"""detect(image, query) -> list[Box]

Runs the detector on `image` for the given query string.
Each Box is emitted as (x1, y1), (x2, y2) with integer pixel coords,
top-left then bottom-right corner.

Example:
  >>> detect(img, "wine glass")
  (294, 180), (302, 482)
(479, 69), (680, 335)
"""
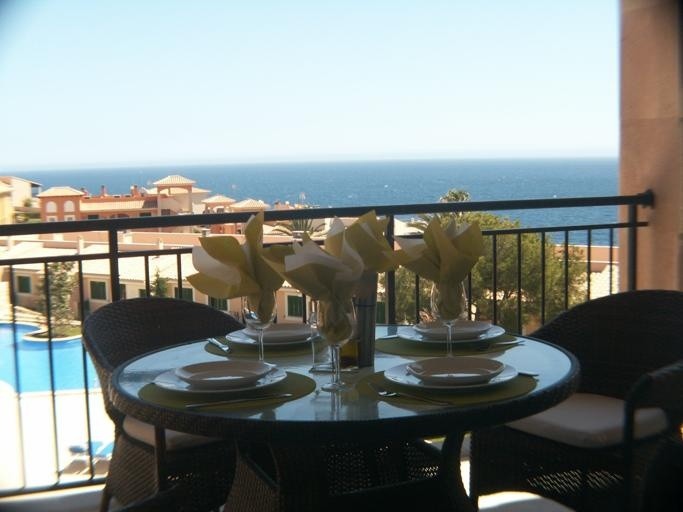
(433, 281), (466, 354)
(241, 288), (277, 359)
(317, 298), (357, 393)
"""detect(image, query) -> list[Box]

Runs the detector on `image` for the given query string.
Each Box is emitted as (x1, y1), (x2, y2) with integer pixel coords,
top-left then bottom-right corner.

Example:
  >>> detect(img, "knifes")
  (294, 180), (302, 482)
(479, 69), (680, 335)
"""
(185, 393), (294, 409)
(208, 337), (232, 355)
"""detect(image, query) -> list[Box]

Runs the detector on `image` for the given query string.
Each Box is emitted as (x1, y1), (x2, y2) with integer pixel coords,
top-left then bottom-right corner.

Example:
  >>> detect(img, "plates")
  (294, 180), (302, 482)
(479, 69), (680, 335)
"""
(227, 323), (316, 346)
(154, 360), (288, 395)
(386, 355), (520, 391)
(397, 320), (505, 344)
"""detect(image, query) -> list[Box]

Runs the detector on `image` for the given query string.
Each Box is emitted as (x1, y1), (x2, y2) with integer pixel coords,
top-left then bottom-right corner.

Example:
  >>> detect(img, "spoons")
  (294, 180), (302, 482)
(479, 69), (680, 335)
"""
(369, 381), (453, 406)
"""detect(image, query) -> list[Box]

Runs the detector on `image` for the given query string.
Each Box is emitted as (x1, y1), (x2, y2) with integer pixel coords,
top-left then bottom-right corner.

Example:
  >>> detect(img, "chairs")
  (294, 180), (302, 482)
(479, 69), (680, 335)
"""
(468, 289), (682, 511)
(78, 297), (247, 512)
